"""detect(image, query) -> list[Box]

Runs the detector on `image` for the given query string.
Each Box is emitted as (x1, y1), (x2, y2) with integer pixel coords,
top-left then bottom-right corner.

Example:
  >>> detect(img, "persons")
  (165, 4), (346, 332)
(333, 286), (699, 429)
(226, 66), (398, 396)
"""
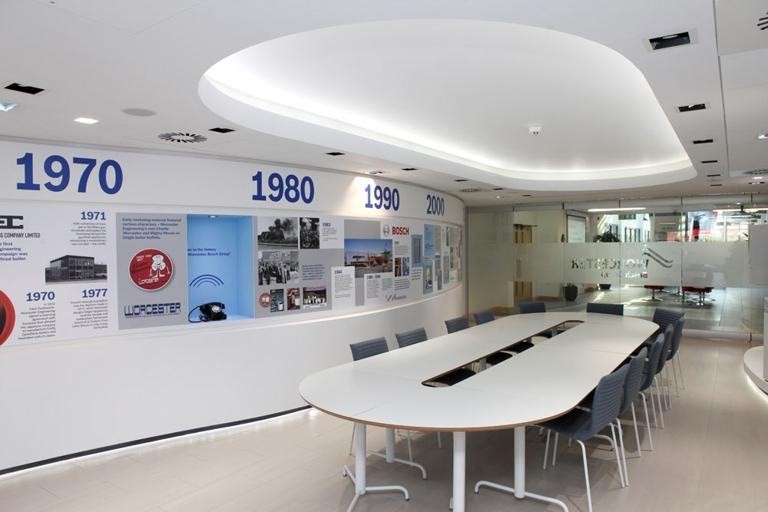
(257, 218), (326, 304)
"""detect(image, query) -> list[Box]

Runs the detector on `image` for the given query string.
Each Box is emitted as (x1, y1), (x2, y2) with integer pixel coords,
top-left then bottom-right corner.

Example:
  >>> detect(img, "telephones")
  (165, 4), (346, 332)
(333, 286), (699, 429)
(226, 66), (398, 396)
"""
(199, 302), (226, 321)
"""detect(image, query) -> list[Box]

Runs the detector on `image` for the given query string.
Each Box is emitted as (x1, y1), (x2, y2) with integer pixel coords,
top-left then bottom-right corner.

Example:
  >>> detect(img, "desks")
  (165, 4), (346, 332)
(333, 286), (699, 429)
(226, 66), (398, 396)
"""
(298, 312), (660, 512)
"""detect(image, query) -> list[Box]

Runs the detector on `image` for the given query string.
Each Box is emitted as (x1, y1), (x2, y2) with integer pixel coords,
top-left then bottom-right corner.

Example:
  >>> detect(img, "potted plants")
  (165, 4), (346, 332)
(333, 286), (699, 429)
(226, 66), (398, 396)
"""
(593, 230), (622, 289)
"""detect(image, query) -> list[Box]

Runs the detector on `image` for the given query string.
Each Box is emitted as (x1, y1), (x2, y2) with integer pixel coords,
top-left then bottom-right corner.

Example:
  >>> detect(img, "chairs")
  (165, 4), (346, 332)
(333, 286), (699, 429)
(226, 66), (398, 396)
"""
(518, 302), (550, 338)
(350, 337), (439, 480)
(445, 315), (512, 365)
(535, 303), (685, 512)
(394, 328), (475, 385)
(473, 311), (534, 352)
(644, 274), (713, 306)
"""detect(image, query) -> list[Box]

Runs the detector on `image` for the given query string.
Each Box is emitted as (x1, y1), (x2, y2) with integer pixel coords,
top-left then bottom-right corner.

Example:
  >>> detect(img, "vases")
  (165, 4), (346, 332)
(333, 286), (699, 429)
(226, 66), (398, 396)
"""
(562, 287), (577, 301)
